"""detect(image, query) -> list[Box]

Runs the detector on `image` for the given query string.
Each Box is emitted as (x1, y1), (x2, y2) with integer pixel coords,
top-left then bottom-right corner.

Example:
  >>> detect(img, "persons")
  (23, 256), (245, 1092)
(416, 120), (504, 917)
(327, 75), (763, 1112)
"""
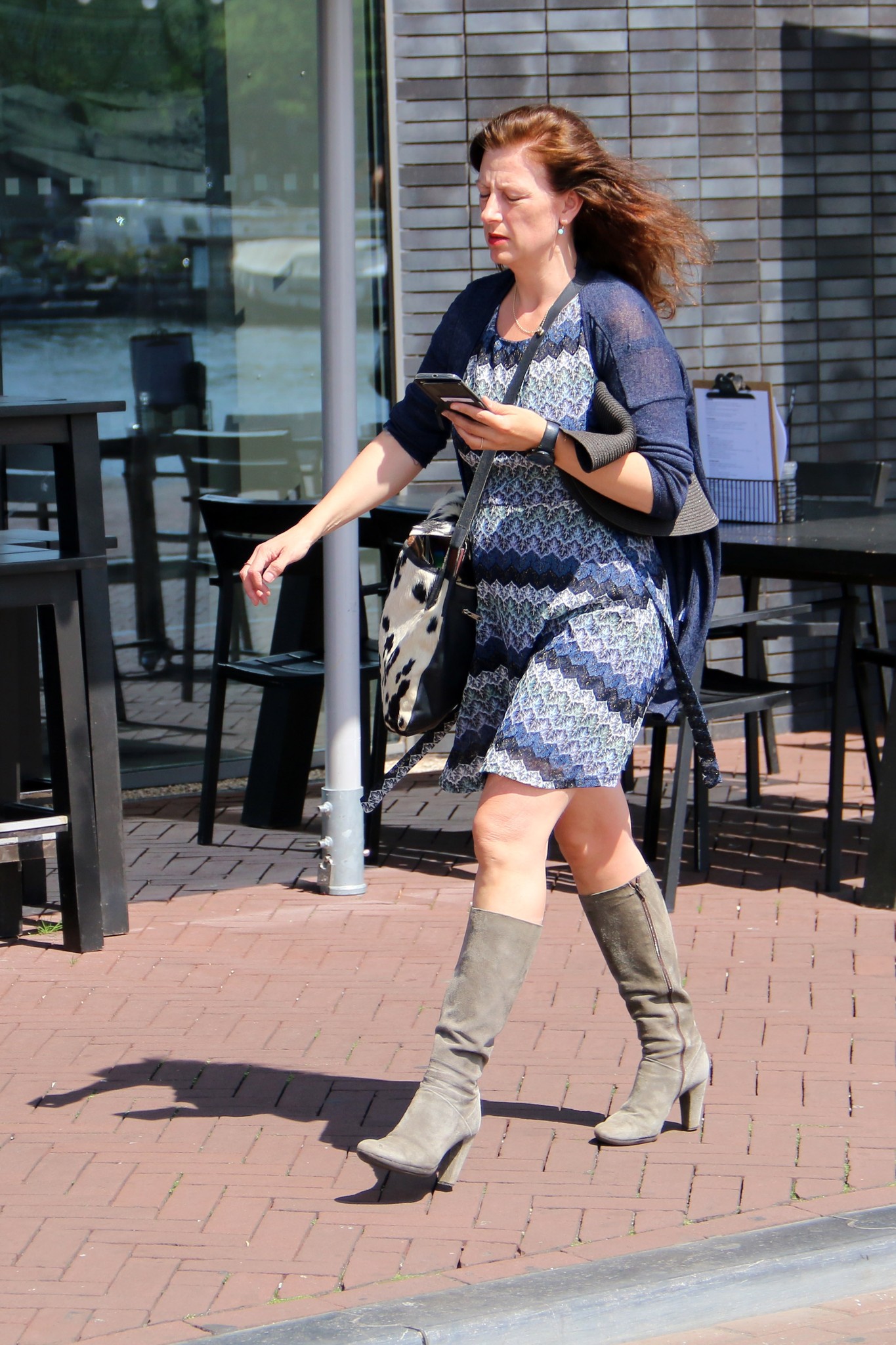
(240, 106), (722, 1185)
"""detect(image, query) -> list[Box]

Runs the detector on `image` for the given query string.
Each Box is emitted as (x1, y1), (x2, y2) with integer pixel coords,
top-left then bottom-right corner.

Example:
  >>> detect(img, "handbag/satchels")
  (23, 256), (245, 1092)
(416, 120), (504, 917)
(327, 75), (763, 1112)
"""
(378, 493), (470, 733)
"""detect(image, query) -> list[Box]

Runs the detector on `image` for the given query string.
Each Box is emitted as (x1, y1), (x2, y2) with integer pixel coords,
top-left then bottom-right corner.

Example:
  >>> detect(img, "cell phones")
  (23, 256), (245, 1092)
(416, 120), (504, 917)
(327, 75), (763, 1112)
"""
(414, 372), (489, 421)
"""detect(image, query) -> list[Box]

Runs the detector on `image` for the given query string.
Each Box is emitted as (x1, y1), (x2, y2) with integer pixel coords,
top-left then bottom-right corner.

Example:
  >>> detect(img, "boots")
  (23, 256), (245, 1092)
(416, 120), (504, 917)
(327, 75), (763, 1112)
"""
(355, 905), (542, 1188)
(578, 867), (710, 1146)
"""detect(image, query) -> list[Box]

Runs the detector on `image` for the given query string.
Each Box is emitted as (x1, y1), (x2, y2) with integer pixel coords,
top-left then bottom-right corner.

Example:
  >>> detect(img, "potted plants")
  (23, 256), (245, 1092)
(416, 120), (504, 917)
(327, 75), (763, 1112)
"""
(42, 235), (192, 412)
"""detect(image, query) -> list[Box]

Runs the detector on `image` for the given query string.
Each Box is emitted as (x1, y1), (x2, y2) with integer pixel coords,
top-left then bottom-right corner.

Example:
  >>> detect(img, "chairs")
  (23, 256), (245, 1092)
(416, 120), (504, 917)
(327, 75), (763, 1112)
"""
(194, 493), (380, 846)
(1, 396), (118, 786)
(167, 428), (309, 703)
(0, 544), (116, 956)
(372, 461), (891, 877)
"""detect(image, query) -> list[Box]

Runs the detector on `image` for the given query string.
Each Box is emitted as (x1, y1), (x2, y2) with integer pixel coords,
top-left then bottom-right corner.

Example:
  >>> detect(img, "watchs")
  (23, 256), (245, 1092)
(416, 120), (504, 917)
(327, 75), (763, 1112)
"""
(526, 418), (561, 466)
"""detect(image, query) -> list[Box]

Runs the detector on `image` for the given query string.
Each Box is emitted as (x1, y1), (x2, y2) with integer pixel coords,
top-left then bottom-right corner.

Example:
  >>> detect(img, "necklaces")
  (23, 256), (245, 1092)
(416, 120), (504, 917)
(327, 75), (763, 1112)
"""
(513, 282), (555, 336)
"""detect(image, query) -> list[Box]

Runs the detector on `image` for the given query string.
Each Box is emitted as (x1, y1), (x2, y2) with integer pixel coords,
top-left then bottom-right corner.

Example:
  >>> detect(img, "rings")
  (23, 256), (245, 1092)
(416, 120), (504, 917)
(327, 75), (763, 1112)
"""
(480, 437), (483, 450)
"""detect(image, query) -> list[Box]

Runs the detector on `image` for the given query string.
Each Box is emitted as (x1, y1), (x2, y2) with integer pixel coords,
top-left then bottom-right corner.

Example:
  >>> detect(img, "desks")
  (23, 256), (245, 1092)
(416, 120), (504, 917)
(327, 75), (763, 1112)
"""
(0, 392), (129, 936)
(239, 489), (896, 910)
(93, 429), (371, 672)
